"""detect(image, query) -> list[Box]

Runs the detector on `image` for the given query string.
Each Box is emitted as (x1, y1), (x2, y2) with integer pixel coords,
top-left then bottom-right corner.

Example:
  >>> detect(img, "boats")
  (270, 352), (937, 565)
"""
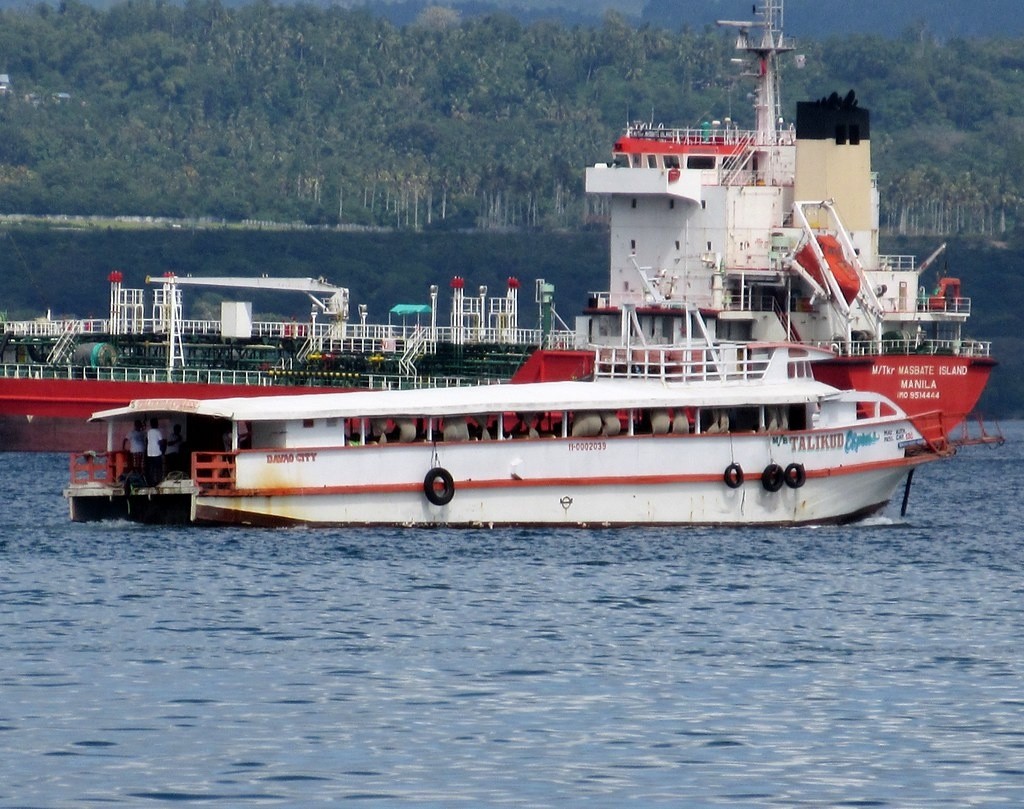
(795, 232), (861, 308)
(1, 1), (1011, 531)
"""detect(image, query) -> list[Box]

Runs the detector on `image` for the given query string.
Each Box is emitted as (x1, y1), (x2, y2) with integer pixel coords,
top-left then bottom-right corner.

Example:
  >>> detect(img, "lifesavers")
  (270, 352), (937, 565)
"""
(723, 463), (745, 488)
(423, 467), (455, 505)
(761, 463), (808, 493)
